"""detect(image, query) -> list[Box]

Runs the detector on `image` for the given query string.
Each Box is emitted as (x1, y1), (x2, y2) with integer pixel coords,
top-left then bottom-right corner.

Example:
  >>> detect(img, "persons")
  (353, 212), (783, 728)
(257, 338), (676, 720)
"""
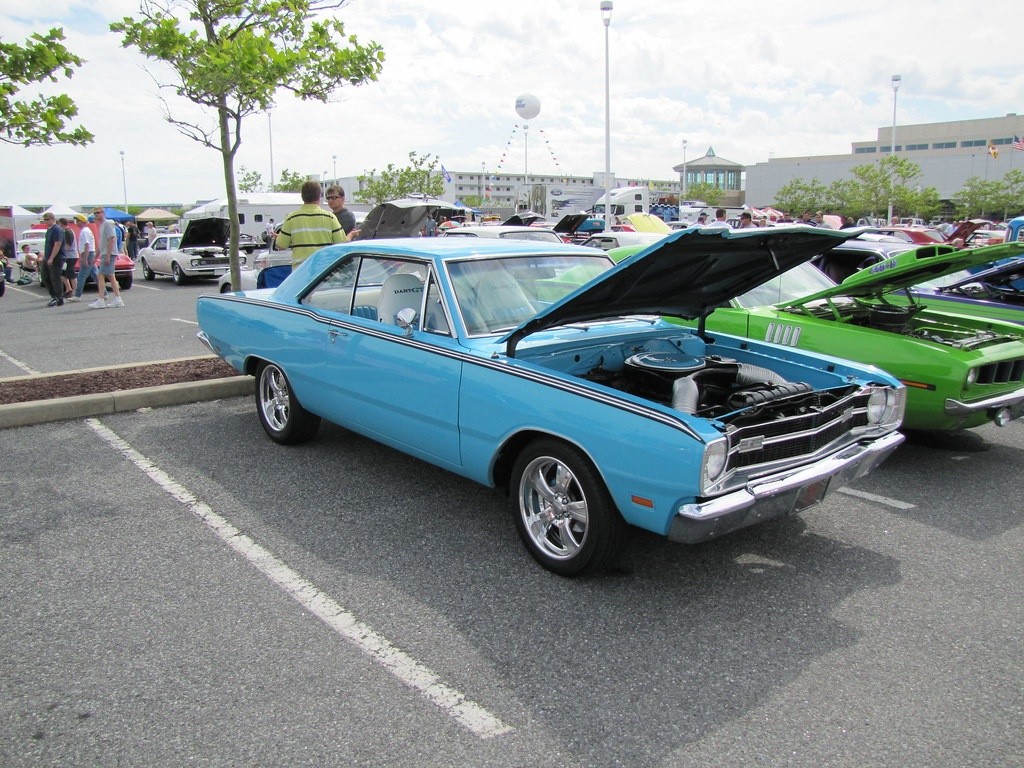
(323, 185), (357, 235)
(813, 210), (833, 229)
(707, 208), (734, 233)
(887, 216), (899, 226)
(906, 219), (913, 227)
(777, 208), (794, 223)
(419, 213), (446, 236)
(144, 221), (157, 247)
(0, 250), (9, 272)
(759, 216), (768, 227)
(114, 220), (140, 260)
(270, 180), (362, 274)
(740, 213), (758, 228)
(58, 218), (79, 299)
(797, 210), (817, 226)
(839, 216), (856, 230)
(681, 212), (709, 228)
(66, 213), (110, 302)
(15, 244), (44, 272)
(40, 212), (64, 307)
(945, 220), (958, 237)
(266, 218), (278, 253)
(88, 207), (125, 309)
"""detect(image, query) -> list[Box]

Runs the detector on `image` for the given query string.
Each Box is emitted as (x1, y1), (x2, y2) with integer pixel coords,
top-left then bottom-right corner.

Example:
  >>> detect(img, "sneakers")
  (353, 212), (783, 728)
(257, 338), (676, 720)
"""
(87, 299), (105, 309)
(107, 299), (125, 308)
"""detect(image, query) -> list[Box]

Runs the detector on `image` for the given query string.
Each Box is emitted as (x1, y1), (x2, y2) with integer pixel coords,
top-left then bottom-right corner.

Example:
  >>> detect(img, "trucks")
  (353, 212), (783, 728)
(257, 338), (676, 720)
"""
(591, 187), (650, 222)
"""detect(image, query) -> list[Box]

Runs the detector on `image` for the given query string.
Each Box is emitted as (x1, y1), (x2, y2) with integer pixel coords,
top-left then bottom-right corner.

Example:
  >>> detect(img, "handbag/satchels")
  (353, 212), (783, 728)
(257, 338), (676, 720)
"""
(17, 275), (32, 284)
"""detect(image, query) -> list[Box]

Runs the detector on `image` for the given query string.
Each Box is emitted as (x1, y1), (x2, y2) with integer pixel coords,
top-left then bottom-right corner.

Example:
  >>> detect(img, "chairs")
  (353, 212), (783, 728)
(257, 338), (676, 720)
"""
(830, 254), (861, 283)
(378, 274), (449, 333)
(475, 271), (536, 327)
(17, 253), (39, 282)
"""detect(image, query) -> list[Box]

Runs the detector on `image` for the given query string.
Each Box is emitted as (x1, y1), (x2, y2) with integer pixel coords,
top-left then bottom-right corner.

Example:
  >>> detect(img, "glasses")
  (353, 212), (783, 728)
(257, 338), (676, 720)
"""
(43, 218), (50, 221)
(326, 195), (341, 201)
(94, 212), (101, 214)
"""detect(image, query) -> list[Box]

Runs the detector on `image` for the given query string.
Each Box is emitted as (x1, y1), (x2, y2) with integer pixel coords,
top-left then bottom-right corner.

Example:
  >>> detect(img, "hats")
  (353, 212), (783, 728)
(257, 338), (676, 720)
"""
(737, 212), (751, 218)
(73, 214), (88, 222)
(146, 222), (153, 226)
(700, 212), (709, 216)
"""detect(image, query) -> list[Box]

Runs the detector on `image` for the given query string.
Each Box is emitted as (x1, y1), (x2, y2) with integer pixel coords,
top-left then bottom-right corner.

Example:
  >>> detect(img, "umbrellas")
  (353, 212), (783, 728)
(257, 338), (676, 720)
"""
(742, 203), (782, 219)
(0, 197), (230, 227)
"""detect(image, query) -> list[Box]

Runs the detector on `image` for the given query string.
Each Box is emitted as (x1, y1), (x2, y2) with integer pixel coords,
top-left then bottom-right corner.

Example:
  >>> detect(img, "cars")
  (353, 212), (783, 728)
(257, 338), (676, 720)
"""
(37, 223), (137, 289)
(195, 222), (907, 579)
(16, 229), (48, 256)
(224, 233), (256, 253)
(216, 213), (1023, 324)
(137, 216), (248, 286)
(536, 240), (1024, 433)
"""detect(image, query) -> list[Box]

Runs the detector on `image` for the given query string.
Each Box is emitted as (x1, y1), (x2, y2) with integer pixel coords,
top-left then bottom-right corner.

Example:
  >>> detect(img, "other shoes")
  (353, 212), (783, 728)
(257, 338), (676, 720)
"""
(62, 290), (73, 298)
(96, 292), (109, 299)
(47, 298), (63, 306)
(66, 296), (81, 302)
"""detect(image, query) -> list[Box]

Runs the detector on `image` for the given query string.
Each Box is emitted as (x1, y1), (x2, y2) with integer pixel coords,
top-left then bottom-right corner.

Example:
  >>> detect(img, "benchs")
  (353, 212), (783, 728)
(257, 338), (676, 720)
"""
(309, 286), (438, 321)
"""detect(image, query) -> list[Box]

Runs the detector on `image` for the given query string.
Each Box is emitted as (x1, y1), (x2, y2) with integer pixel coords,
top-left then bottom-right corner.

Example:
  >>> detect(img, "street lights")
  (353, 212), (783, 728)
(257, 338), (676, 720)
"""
(268, 112), (274, 192)
(524, 125), (529, 184)
(323, 171), (327, 203)
(332, 156), (337, 185)
(682, 140), (687, 194)
(120, 151), (128, 213)
(482, 162), (485, 199)
(888, 75), (901, 226)
(600, 1), (613, 231)
